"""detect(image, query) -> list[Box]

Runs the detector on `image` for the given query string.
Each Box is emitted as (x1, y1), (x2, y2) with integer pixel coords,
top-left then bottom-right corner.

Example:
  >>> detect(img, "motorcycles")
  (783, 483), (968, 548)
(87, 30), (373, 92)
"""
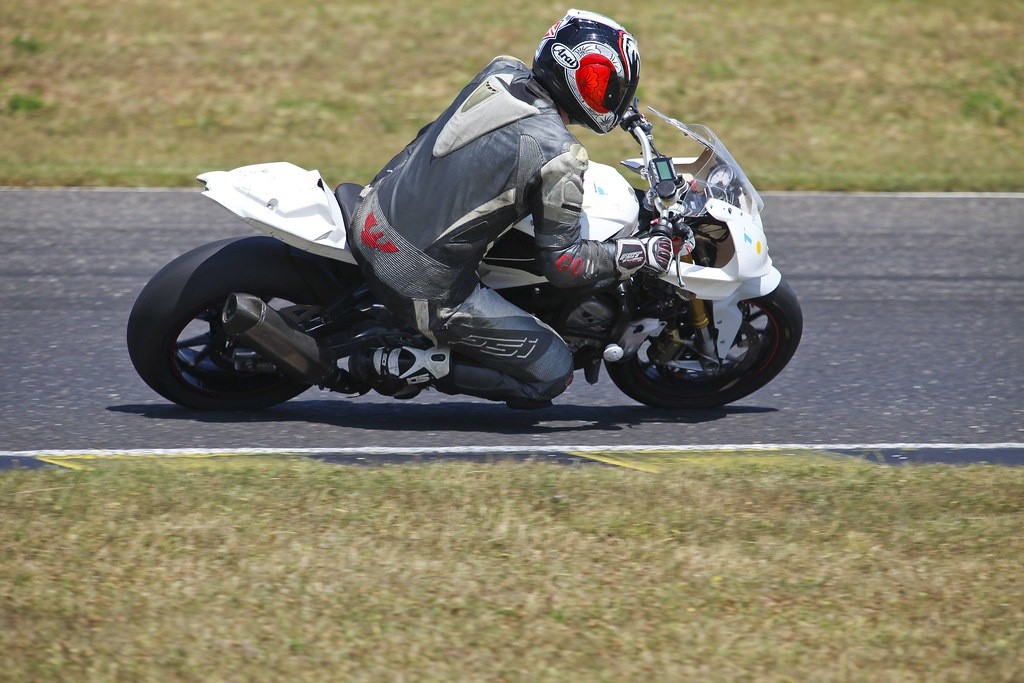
(127, 98), (804, 414)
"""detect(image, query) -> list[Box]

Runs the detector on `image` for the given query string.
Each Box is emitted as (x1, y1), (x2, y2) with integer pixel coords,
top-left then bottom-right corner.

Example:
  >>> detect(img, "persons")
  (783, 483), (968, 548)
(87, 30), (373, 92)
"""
(348, 10), (670, 406)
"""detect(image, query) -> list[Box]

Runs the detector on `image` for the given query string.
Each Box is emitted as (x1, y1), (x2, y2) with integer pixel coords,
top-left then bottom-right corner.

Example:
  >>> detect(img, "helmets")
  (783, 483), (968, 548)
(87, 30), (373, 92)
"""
(532, 8), (642, 135)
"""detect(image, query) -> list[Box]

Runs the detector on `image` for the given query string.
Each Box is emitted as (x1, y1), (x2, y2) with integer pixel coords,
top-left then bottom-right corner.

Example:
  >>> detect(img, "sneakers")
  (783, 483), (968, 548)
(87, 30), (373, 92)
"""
(374, 343), (453, 398)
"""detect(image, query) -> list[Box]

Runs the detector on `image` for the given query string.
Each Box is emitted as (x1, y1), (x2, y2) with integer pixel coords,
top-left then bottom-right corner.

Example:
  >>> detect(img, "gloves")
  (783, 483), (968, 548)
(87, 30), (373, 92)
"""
(619, 104), (642, 131)
(608, 235), (675, 281)
